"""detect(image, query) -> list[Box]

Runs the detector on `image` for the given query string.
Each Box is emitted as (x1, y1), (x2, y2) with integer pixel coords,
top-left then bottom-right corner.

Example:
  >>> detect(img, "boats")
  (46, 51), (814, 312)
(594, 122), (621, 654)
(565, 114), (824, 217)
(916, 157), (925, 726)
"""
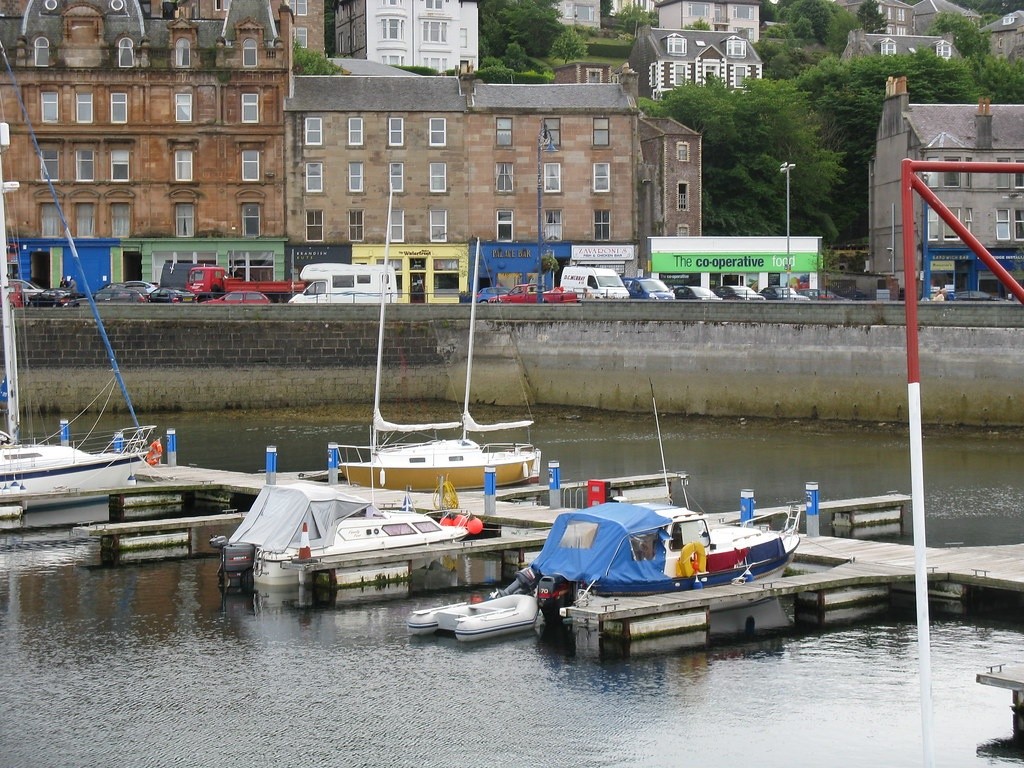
(489, 501), (800, 626)
(405, 594), (539, 641)
(207, 482), (475, 585)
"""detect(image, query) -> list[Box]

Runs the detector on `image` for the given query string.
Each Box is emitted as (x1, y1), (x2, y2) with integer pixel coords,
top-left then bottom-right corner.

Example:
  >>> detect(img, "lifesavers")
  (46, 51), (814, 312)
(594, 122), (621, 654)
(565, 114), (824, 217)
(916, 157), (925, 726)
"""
(679, 541), (707, 577)
(146, 441), (162, 465)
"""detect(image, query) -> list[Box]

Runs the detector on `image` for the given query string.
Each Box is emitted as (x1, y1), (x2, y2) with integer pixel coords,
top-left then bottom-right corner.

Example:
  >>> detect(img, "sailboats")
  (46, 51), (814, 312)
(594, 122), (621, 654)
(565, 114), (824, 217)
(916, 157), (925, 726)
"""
(0, 36), (158, 503)
(336, 182), (542, 493)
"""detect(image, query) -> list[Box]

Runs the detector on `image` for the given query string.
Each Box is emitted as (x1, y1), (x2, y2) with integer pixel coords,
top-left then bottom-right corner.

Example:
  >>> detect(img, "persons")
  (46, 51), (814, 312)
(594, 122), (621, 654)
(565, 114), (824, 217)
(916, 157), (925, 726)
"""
(69, 278), (77, 293)
(60, 277), (68, 288)
(932, 288), (954, 301)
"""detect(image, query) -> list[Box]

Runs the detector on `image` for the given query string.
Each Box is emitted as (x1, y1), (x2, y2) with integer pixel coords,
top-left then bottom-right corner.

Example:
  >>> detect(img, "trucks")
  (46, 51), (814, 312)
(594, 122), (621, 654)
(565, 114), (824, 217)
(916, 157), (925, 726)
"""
(185, 266), (307, 304)
(288, 262), (398, 303)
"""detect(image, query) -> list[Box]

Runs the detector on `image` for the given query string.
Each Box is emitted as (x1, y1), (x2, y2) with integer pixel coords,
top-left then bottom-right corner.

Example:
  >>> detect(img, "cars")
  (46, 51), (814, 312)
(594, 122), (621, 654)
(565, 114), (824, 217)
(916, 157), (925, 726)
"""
(0, 279), (199, 307)
(673, 286), (723, 301)
(201, 289), (272, 304)
(757, 286), (810, 301)
(476, 287), (511, 303)
(712, 285), (765, 301)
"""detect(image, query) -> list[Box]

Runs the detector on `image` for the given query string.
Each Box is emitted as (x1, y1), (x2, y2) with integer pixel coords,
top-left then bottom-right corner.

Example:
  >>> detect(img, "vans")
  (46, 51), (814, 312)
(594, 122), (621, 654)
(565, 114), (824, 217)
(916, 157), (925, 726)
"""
(559, 266), (631, 300)
(619, 277), (676, 300)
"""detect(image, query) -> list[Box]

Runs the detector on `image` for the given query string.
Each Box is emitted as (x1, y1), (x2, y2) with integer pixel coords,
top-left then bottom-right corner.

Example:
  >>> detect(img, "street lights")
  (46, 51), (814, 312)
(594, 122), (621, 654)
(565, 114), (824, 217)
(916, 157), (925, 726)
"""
(779, 162), (797, 300)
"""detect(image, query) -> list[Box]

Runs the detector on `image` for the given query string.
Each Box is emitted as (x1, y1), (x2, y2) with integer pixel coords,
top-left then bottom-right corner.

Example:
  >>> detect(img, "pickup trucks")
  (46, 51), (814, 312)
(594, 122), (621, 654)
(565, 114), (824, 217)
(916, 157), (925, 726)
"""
(488, 283), (578, 303)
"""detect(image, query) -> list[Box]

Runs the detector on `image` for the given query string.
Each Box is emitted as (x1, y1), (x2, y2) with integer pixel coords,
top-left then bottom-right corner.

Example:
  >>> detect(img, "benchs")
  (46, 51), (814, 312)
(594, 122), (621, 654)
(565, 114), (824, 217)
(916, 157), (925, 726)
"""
(470, 606), (506, 610)
